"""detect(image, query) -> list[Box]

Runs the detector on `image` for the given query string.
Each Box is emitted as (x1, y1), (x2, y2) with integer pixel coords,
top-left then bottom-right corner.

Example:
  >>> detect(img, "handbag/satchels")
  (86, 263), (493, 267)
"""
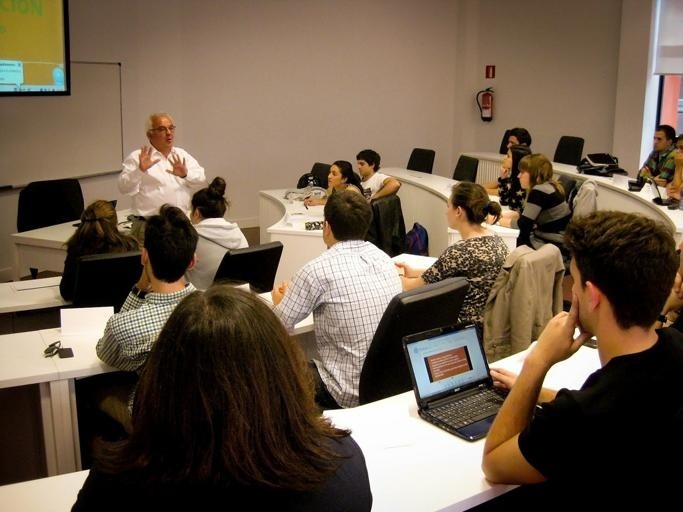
(578, 153), (628, 178)
(406, 223), (429, 256)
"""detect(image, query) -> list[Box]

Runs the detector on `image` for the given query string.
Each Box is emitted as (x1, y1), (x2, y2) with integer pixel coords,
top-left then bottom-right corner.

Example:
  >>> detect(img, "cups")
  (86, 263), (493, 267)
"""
(310, 191), (321, 204)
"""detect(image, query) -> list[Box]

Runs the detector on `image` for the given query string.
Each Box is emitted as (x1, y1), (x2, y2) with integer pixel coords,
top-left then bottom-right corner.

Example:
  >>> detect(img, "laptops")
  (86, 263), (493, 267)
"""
(642, 174), (677, 205)
(73, 200), (119, 229)
(403, 318), (514, 442)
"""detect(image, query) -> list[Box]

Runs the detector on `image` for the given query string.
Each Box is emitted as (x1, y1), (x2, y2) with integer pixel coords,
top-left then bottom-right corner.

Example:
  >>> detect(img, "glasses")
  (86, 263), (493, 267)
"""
(149, 125), (176, 133)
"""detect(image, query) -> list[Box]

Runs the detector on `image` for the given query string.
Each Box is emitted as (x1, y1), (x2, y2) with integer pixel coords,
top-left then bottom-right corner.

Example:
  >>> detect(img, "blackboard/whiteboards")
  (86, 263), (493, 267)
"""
(0, 60), (124, 193)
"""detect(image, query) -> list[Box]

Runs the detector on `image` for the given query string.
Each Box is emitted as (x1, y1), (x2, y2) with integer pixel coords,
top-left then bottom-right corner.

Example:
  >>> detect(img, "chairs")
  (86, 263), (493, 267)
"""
(311, 162), (332, 190)
(452, 155), (479, 184)
(407, 147), (437, 174)
(553, 136), (585, 166)
(17, 178), (84, 234)
(72, 242), (283, 315)
(309, 277), (470, 410)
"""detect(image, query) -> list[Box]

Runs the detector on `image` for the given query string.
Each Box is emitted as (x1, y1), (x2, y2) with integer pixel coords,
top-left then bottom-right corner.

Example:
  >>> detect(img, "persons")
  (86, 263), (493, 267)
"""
(60, 199), (139, 301)
(75, 204), (204, 460)
(117, 112), (205, 250)
(70, 283), (373, 512)
(185, 177), (272, 307)
(482, 210), (683, 512)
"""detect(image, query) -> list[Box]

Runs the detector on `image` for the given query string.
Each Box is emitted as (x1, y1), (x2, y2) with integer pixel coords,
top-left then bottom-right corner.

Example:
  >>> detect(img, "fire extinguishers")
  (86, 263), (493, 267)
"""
(476, 86), (494, 121)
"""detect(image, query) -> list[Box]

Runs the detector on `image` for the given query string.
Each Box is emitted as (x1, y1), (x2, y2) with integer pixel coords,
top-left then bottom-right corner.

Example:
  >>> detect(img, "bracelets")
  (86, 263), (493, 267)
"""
(132, 285), (147, 300)
(181, 174), (187, 178)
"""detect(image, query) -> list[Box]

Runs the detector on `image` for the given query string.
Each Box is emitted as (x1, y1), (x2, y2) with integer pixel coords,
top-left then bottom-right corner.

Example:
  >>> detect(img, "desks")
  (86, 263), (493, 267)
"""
(550, 161), (683, 251)
(9, 208), (137, 282)
(258, 187), (328, 291)
(0, 276), (72, 336)
(392, 253), (439, 276)
(377, 167), (520, 259)
(462, 151), (508, 185)
(1, 291), (603, 510)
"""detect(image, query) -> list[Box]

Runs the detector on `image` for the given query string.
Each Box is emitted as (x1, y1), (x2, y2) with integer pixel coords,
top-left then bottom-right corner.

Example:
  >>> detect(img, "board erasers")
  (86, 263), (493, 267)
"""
(0, 185), (13, 191)
